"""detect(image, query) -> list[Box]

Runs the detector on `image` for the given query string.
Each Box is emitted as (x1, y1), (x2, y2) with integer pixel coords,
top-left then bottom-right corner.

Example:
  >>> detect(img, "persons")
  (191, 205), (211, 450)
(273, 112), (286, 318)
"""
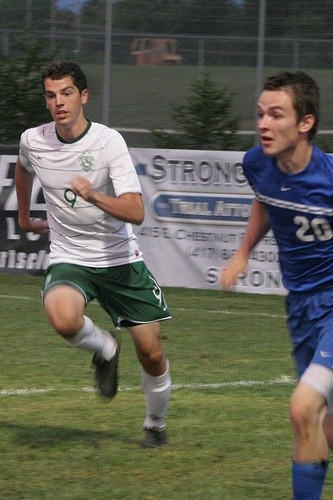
(214, 69), (331, 500)
(14, 61), (178, 448)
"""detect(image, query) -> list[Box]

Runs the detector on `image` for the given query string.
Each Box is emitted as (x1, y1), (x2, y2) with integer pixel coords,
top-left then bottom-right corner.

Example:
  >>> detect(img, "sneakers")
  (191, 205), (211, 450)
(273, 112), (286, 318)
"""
(92, 329), (119, 398)
(140, 424), (168, 452)
